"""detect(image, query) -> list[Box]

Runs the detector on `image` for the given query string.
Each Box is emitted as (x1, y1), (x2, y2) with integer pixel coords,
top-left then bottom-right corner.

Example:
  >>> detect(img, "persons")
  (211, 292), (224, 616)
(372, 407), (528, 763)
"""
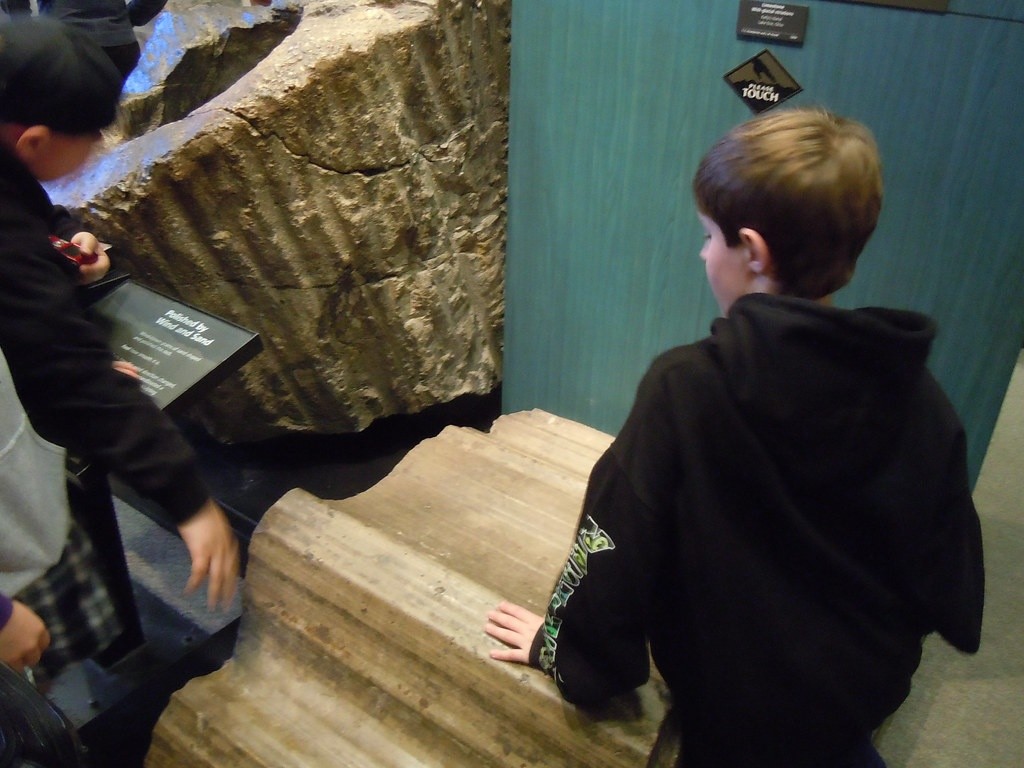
(0, 0), (238, 768)
(485, 106), (984, 768)
